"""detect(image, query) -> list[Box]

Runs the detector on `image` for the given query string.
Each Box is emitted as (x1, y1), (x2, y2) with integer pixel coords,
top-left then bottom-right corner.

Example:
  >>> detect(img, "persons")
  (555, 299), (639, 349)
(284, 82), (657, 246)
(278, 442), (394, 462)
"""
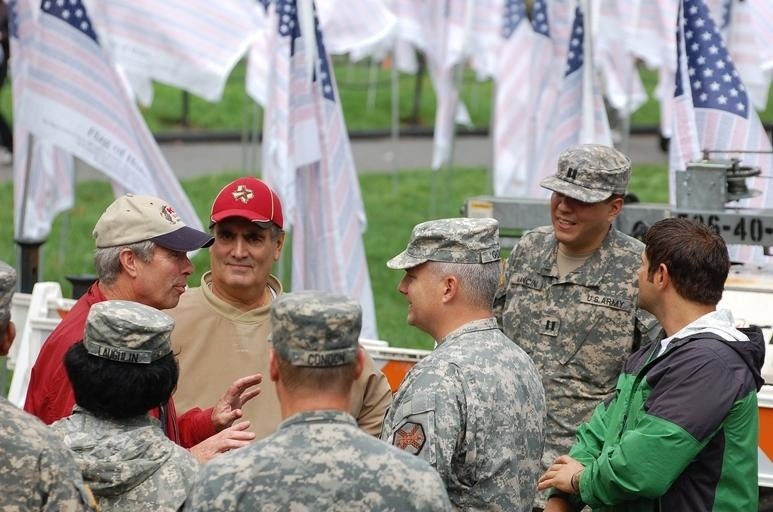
(376, 216), (549, 512)
(23, 191), (264, 462)
(45, 297), (200, 511)
(160, 173), (393, 441)
(183, 290), (453, 511)
(0, 258), (101, 511)
(493, 142), (662, 512)
(536, 215), (767, 512)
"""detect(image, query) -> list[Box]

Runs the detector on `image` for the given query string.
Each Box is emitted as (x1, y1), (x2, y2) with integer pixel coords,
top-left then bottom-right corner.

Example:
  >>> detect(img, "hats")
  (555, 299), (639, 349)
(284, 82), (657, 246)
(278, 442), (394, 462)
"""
(83, 301), (174, 363)
(540, 145), (631, 204)
(0, 262), (18, 325)
(92, 193), (215, 253)
(386, 217), (501, 269)
(266, 290), (363, 366)
(78, 426), (197, 512)
(208, 179), (282, 230)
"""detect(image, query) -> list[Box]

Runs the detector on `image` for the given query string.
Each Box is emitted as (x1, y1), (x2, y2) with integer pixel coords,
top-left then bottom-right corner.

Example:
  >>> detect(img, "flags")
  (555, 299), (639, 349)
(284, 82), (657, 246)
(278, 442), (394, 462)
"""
(7, 1), (772, 339)
